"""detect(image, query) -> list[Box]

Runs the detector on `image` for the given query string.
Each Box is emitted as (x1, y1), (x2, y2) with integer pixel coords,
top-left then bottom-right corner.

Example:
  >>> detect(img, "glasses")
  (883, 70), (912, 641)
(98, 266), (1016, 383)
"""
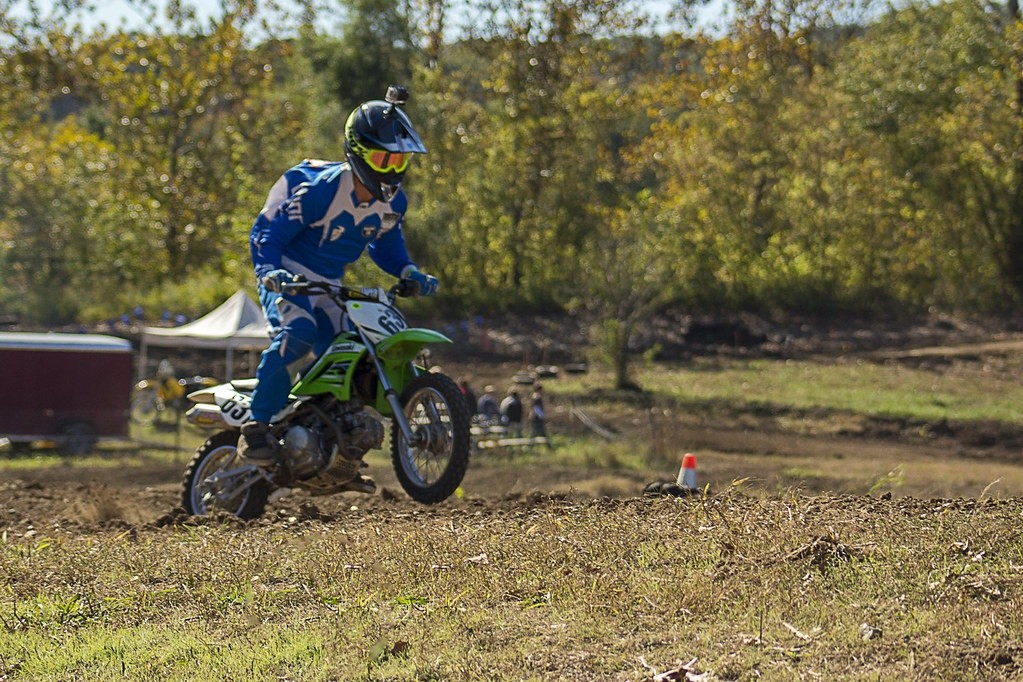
(365, 149), (413, 173)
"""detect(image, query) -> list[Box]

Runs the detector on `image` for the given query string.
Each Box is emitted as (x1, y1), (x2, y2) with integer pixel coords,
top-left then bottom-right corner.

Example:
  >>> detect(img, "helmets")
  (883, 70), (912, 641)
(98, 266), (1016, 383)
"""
(344, 100), (427, 204)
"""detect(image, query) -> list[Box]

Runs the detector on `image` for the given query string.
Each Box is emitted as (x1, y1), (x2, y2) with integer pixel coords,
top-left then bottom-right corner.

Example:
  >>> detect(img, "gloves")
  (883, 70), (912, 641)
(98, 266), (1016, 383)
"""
(261, 269), (296, 295)
(402, 265), (439, 297)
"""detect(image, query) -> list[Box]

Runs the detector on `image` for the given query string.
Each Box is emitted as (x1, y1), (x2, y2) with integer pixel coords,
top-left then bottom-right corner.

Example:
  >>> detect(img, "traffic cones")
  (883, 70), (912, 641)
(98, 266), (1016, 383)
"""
(675, 453), (696, 492)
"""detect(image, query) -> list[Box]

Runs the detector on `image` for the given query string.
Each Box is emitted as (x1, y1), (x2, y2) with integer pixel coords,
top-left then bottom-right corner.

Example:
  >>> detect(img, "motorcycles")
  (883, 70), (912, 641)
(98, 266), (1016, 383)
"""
(179, 272), (473, 523)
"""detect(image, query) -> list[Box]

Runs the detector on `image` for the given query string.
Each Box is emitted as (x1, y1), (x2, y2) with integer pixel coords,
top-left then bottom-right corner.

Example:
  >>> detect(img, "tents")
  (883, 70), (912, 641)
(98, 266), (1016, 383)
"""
(138, 290), (276, 386)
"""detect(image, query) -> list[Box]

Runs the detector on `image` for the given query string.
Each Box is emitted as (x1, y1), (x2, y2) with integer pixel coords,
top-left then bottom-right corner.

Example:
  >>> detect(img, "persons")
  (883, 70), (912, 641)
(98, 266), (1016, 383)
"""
(457, 377), (478, 415)
(236, 100), (441, 465)
(499, 385), (524, 461)
(526, 382), (553, 448)
(477, 384), (501, 425)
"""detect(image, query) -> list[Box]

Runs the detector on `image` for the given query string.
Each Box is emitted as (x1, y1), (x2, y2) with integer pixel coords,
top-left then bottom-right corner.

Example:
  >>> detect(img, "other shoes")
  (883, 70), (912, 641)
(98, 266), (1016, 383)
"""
(235, 419), (276, 468)
